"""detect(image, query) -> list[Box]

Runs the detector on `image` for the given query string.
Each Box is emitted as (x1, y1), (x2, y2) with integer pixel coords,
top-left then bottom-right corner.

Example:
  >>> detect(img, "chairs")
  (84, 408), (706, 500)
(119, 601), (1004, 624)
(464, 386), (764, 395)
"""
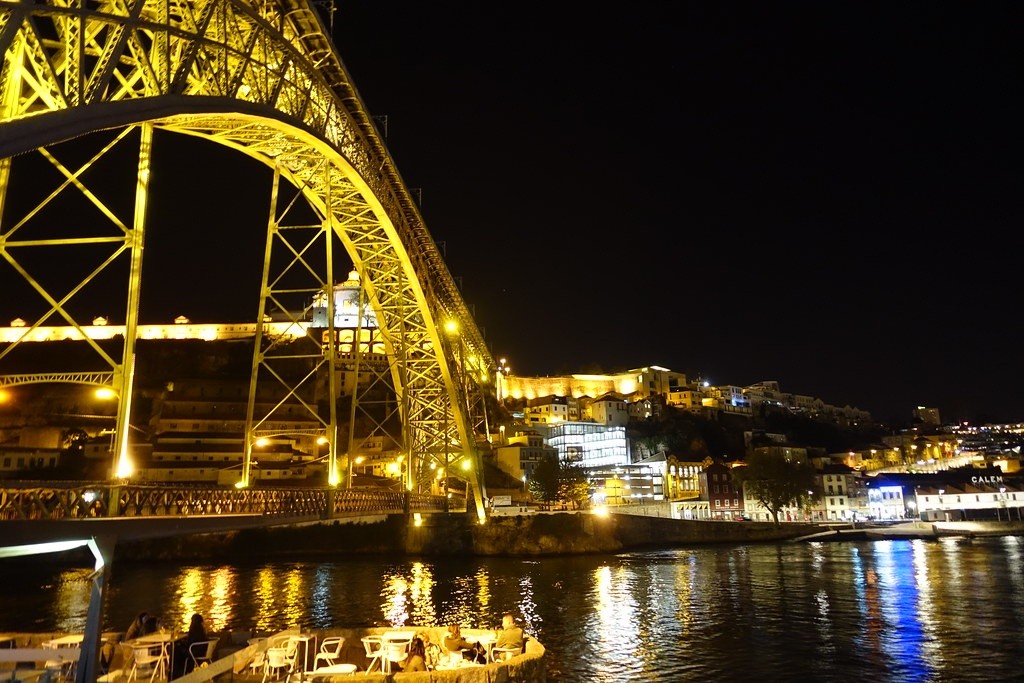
(491, 646), (523, 663)
(127, 642), (165, 683)
(445, 647), (479, 668)
(383, 640), (410, 673)
(360, 636), (393, 675)
(262, 646), (297, 683)
(313, 636), (346, 672)
(35, 641), (76, 682)
(189, 636), (221, 676)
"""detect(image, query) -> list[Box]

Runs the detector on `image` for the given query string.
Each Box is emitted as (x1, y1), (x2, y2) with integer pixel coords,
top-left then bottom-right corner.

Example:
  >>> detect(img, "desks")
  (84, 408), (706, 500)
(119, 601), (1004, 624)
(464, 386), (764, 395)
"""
(317, 664), (357, 674)
(50, 634), (85, 681)
(136, 632), (187, 678)
(384, 631), (413, 672)
(465, 636), (500, 666)
(290, 632), (319, 683)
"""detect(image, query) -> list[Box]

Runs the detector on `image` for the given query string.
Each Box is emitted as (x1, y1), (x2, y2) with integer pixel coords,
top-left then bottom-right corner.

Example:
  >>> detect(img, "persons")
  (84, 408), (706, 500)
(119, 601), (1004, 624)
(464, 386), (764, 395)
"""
(403, 639), (426, 672)
(444, 623), (486, 664)
(168, 614), (208, 683)
(125, 613), (150, 641)
(412, 632), (437, 671)
(138, 618), (158, 636)
(488, 614), (523, 663)
